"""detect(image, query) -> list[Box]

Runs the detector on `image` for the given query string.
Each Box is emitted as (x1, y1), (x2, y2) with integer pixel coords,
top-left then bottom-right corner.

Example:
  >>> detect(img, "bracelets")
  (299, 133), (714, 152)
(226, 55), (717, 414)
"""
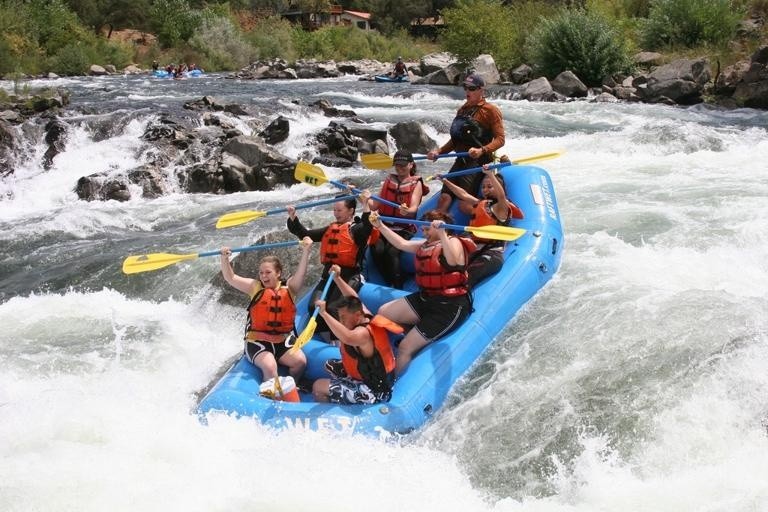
(481, 147), (487, 155)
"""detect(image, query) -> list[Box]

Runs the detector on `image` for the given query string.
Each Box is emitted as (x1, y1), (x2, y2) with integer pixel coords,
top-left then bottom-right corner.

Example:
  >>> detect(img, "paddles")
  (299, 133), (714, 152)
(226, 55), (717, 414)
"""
(122, 240), (301, 275)
(288, 271), (336, 355)
(376, 216), (527, 242)
(360, 151), (469, 169)
(294, 161), (401, 210)
(215, 193), (360, 229)
(425, 153), (559, 183)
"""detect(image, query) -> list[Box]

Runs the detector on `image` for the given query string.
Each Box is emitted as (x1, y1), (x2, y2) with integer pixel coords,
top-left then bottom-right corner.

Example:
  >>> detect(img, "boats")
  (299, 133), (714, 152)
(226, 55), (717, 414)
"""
(374, 75), (410, 83)
(193, 163), (566, 437)
(153, 69), (203, 79)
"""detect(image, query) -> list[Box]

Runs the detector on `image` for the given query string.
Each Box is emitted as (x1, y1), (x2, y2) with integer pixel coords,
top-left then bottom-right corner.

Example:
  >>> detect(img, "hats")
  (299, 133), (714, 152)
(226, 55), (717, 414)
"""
(392, 149), (415, 167)
(458, 74), (485, 88)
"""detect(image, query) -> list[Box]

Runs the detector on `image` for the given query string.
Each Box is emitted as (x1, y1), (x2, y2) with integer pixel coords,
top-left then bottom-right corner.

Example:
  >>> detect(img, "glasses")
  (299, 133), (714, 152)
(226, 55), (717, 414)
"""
(463, 85), (477, 92)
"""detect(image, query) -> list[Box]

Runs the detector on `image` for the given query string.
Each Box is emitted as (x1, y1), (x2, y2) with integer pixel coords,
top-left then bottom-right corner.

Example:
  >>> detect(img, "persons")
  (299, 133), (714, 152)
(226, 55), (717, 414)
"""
(287, 189), (374, 345)
(394, 56), (409, 79)
(152, 60), (206, 76)
(368, 209), (479, 381)
(434, 164), (523, 287)
(425, 77), (507, 217)
(310, 264), (404, 405)
(221, 235), (313, 390)
(347, 150), (431, 290)
